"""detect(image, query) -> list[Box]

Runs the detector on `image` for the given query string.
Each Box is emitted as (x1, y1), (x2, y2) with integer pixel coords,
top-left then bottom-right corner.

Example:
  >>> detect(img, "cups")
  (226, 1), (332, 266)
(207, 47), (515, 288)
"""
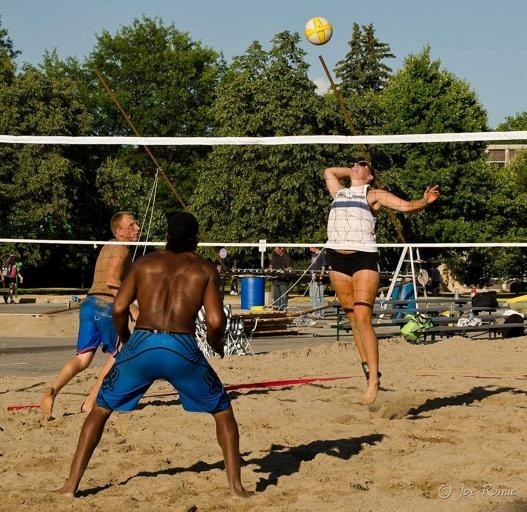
(449, 306), (455, 319)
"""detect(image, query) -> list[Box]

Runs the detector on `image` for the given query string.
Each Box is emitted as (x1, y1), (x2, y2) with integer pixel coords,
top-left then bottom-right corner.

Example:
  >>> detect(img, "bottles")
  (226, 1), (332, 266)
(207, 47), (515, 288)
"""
(454, 287), (459, 300)
(468, 309), (474, 320)
(471, 287), (476, 299)
(380, 290), (385, 310)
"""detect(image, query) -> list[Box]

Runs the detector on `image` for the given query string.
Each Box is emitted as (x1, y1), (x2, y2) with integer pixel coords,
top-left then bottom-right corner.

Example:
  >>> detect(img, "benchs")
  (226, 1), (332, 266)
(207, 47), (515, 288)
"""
(329, 298), (526, 342)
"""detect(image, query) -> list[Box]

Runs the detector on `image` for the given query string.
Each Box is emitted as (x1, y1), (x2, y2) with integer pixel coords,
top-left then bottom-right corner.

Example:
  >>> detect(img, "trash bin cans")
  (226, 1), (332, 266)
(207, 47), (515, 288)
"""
(390, 281), (417, 320)
(239, 275), (265, 310)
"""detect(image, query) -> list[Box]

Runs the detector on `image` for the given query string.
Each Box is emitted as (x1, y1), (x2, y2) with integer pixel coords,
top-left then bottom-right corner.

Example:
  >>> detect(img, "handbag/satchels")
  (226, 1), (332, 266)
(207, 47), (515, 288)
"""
(472, 290), (498, 307)
(400, 313), (433, 344)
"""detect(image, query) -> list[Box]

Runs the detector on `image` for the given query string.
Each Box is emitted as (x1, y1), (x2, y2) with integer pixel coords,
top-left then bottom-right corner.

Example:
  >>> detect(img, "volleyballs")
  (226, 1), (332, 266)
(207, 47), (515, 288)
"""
(305, 16), (333, 45)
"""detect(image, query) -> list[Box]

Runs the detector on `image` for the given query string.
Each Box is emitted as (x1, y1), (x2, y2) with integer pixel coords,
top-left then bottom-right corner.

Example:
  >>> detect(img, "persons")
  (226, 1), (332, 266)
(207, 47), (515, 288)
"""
(304, 246), (330, 319)
(266, 244), (296, 310)
(322, 160), (443, 407)
(0, 257), (4, 287)
(5, 253), (18, 304)
(48, 210), (259, 499)
(40, 211), (141, 418)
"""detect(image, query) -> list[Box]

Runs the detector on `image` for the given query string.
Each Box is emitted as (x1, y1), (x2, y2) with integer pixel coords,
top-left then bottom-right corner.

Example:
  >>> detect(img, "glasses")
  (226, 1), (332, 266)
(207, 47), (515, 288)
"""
(351, 162), (371, 170)
